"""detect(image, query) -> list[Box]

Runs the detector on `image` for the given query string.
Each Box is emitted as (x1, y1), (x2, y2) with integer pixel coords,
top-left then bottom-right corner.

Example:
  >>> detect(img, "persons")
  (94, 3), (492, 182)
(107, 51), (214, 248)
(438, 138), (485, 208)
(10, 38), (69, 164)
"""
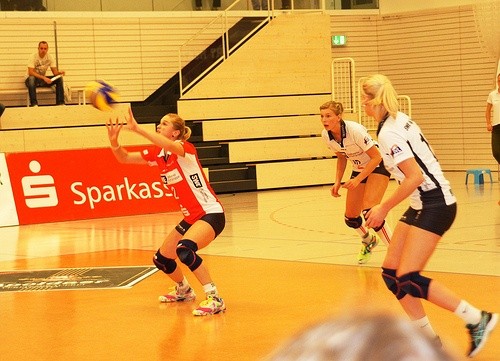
(360, 73), (497, 359)
(320, 101), (393, 263)
(106, 108), (226, 315)
(486, 74), (500, 166)
(24, 41), (65, 107)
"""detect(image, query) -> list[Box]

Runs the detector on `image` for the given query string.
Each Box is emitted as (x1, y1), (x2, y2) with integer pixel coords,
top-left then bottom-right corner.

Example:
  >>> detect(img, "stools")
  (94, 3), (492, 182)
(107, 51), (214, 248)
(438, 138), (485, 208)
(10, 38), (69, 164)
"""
(465, 168), (493, 184)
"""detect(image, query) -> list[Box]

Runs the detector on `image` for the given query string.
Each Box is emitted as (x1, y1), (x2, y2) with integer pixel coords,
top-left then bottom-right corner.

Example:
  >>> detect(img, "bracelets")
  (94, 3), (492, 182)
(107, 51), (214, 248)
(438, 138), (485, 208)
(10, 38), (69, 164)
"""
(113, 146), (120, 150)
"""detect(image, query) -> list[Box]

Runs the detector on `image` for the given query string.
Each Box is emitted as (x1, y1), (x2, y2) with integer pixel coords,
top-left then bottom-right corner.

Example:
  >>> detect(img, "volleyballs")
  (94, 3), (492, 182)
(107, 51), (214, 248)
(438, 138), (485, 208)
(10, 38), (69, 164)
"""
(87, 80), (120, 111)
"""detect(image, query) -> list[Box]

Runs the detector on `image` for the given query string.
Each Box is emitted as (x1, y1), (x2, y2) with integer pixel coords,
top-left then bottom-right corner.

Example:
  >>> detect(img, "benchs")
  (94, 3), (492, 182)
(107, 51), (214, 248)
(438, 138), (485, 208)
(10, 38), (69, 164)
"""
(0, 87), (359, 190)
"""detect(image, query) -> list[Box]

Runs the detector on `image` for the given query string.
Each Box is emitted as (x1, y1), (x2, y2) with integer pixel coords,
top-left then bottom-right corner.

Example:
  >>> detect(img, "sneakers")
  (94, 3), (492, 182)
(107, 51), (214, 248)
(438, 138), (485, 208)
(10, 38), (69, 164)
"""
(465, 310), (499, 358)
(358, 235), (380, 264)
(192, 295), (226, 316)
(430, 335), (445, 354)
(159, 285), (195, 303)
